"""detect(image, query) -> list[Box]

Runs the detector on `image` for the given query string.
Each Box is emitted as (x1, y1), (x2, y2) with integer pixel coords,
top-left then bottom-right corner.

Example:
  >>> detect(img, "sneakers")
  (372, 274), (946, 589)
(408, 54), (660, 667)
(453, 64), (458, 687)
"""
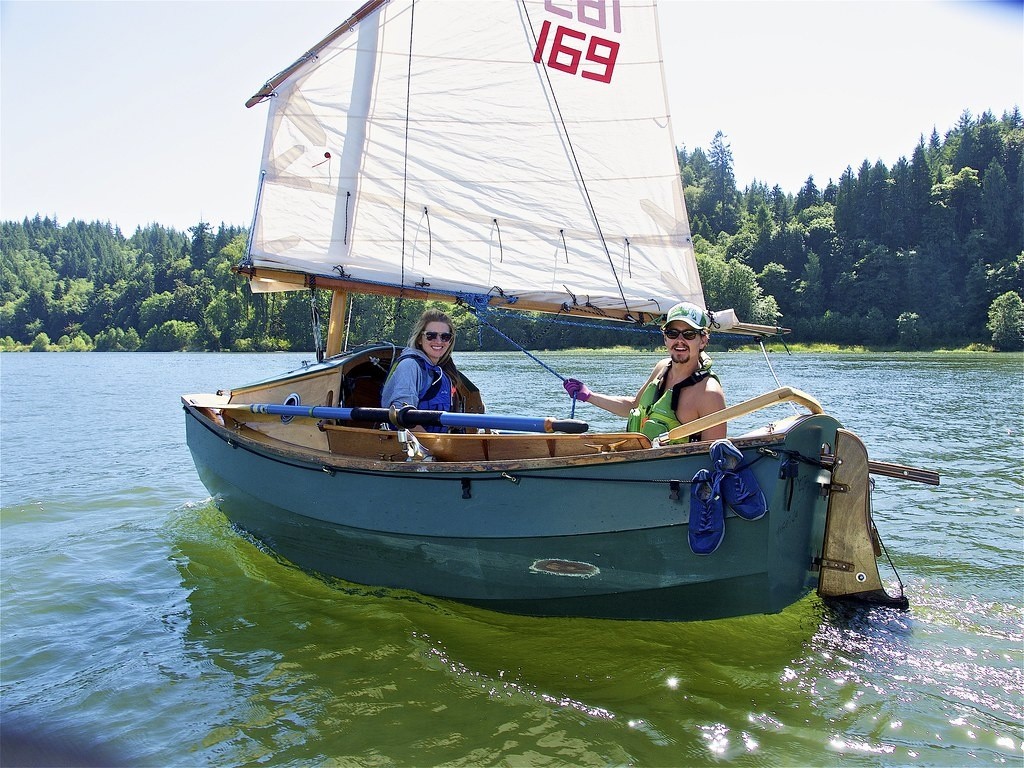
(708, 438), (767, 522)
(687, 469), (727, 556)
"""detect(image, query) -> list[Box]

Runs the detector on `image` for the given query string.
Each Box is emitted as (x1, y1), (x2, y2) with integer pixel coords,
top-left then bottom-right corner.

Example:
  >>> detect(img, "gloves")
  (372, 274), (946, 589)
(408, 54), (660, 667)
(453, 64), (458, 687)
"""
(563, 378), (592, 402)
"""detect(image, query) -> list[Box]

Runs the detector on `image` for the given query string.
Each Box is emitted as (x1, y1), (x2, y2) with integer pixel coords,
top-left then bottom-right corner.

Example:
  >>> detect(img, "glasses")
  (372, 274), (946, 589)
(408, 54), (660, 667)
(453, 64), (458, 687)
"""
(664, 328), (701, 340)
(421, 330), (454, 342)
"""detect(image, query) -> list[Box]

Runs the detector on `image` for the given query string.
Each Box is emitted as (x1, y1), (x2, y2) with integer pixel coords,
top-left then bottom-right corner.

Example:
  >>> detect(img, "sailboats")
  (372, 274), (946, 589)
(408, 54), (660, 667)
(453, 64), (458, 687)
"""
(179, 0), (941, 625)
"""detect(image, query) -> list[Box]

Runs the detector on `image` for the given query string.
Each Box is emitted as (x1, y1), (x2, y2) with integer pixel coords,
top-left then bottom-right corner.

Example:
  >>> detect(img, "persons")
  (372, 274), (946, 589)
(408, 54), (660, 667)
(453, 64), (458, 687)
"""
(563, 302), (726, 446)
(378, 308), (463, 433)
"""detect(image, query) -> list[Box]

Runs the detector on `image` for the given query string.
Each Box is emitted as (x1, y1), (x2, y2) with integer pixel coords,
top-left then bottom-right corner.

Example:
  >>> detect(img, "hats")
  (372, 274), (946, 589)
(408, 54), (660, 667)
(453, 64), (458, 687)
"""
(662, 302), (706, 329)
